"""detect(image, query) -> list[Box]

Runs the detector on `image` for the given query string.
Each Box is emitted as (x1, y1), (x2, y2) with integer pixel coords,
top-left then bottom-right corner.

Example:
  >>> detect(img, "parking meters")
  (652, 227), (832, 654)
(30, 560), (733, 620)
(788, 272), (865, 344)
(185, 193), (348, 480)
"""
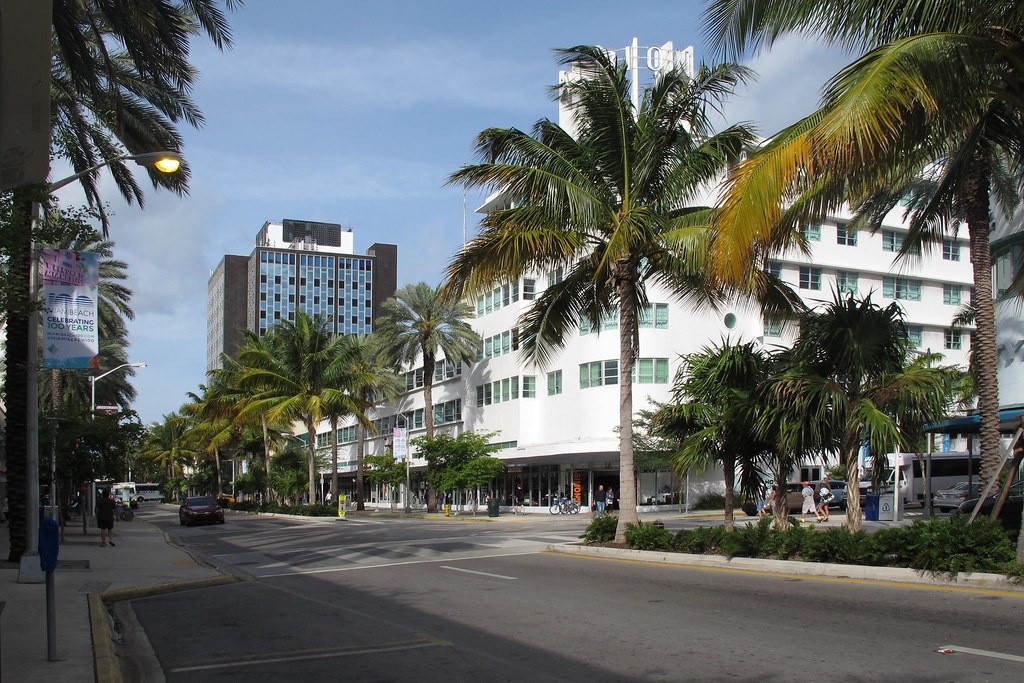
(36, 517), (60, 661)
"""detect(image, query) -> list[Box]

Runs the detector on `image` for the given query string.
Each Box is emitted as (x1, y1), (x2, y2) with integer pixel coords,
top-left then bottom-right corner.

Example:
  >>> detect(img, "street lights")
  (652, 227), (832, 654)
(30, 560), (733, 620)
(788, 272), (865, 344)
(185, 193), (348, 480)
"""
(376, 405), (413, 513)
(18, 150), (184, 584)
(281, 433), (307, 449)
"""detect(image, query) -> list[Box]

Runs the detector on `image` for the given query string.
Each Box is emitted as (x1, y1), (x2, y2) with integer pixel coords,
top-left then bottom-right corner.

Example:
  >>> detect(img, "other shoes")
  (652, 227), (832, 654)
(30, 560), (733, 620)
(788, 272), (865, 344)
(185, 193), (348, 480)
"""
(823, 516), (828, 521)
(109, 542), (117, 547)
(101, 542), (107, 547)
(817, 518), (822, 523)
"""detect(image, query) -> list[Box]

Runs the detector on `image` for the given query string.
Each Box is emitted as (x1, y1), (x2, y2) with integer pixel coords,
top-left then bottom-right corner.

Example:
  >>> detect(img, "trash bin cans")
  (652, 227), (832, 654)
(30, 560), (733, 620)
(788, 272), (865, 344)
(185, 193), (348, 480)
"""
(487, 498), (500, 517)
(866, 485), (904, 521)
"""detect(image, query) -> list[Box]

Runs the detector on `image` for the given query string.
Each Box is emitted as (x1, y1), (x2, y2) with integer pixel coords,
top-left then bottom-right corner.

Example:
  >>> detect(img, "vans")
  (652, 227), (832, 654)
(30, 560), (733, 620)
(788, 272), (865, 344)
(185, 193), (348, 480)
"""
(782, 483), (821, 516)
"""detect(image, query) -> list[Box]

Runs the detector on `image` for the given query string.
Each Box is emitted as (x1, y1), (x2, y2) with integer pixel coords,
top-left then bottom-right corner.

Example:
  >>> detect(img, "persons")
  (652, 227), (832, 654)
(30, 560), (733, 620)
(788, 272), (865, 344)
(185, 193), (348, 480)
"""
(95, 490), (116, 547)
(800, 481), (822, 523)
(594, 485), (606, 518)
(755, 485), (778, 522)
(515, 485), (524, 515)
(2, 494), (8, 520)
(815, 477), (831, 522)
(325, 491), (332, 504)
(605, 487), (614, 518)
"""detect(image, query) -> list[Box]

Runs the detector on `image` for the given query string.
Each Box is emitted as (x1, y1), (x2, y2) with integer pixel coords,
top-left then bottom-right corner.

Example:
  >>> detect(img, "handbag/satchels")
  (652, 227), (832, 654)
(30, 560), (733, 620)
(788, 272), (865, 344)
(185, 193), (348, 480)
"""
(819, 487), (835, 504)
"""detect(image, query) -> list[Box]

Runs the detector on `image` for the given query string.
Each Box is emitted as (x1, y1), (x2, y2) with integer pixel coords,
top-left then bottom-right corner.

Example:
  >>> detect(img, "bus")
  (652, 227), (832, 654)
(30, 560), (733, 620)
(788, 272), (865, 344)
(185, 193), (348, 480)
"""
(134, 483), (167, 502)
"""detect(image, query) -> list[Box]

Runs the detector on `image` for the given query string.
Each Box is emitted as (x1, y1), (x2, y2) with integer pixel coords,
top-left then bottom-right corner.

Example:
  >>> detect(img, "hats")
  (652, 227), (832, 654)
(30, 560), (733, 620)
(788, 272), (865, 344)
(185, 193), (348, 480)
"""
(800, 481), (810, 486)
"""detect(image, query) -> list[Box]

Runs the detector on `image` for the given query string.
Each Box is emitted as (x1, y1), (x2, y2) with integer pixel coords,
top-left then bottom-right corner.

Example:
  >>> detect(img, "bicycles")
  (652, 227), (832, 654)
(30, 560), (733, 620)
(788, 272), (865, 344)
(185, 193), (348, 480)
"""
(549, 497), (582, 515)
(111, 498), (135, 521)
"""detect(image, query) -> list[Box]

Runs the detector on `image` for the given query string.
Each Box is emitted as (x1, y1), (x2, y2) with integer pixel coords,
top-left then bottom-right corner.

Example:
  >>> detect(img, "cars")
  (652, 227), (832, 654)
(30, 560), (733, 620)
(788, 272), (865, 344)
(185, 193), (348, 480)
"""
(933, 482), (986, 514)
(959, 480), (1024, 522)
(179, 496), (226, 526)
(812, 480), (848, 510)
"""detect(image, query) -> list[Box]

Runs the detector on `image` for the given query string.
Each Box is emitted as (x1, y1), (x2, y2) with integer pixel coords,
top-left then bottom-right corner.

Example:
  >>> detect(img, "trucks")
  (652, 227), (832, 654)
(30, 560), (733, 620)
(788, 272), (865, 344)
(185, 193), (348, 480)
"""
(858, 453), (983, 508)
(111, 481), (141, 511)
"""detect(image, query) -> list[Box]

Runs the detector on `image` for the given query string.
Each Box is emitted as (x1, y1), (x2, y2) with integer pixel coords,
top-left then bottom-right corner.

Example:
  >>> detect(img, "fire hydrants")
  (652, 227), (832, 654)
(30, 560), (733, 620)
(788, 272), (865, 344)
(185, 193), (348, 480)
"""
(444, 505), (451, 517)
